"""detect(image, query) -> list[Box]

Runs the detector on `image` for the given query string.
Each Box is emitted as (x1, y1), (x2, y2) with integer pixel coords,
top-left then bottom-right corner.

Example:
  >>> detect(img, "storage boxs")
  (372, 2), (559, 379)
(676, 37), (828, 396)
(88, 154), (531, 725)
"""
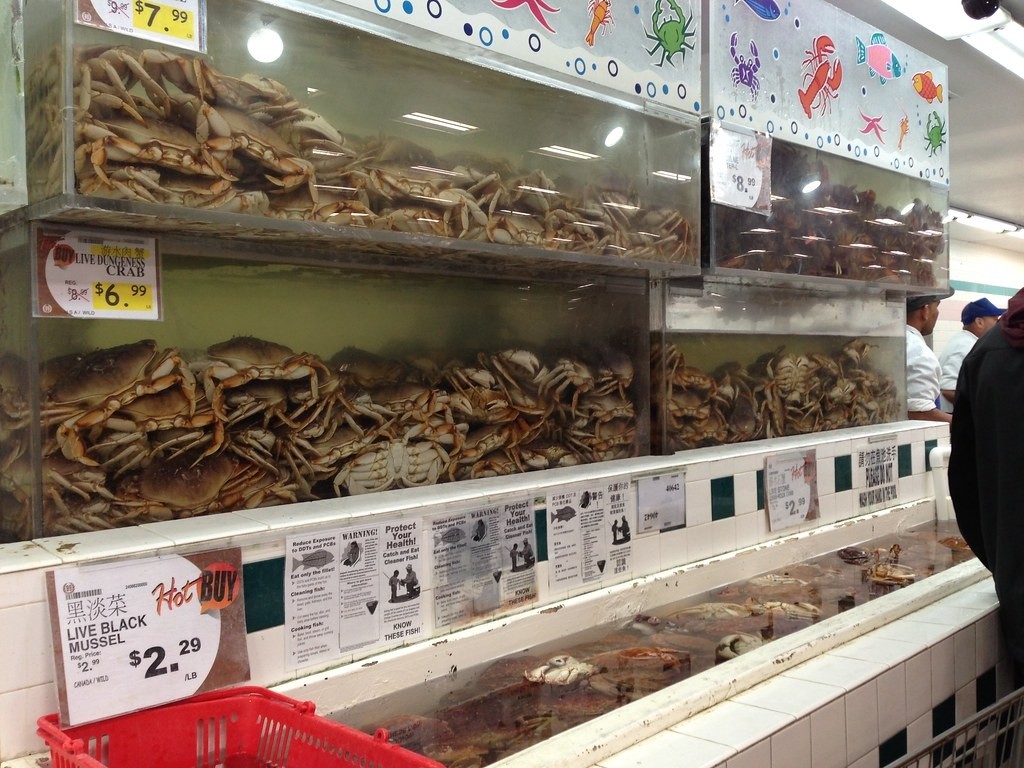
(35, 682), (446, 768)
(0, 0), (951, 540)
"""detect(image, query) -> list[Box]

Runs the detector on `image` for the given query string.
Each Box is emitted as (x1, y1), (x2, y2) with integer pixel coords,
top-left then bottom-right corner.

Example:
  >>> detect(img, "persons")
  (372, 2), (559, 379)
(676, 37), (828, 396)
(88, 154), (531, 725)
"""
(509, 538), (535, 571)
(906, 278), (1024, 682)
(387, 564), (418, 602)
(612, 515), (629, 543)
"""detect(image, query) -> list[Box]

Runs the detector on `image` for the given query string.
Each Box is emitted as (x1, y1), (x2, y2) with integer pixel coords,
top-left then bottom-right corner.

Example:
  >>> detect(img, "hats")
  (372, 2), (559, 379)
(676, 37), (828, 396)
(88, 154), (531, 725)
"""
(907, 285), (955, 312)
(961, 298), (1008, 325)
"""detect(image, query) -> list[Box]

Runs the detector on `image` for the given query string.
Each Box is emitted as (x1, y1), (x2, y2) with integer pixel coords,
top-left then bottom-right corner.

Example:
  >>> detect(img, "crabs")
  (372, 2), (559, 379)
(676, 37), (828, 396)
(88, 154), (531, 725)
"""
(22, 44), (692, 263)
(713, 143), (944, 287)
(0, 335), (642, 540)
(649, 338), (901, 451)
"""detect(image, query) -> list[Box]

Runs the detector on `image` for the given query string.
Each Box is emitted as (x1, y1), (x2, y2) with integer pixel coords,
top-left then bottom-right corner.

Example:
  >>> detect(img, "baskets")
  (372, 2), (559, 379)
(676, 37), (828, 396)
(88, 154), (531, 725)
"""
(37, 685), (450, 768)
